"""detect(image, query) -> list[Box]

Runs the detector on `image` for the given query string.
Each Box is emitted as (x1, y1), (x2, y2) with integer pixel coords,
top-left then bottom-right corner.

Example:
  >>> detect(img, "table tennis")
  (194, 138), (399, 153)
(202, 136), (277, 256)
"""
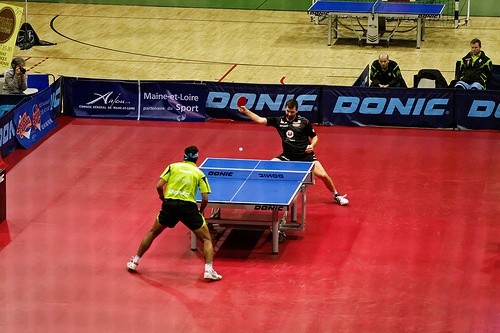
(238, 147), (243, 151)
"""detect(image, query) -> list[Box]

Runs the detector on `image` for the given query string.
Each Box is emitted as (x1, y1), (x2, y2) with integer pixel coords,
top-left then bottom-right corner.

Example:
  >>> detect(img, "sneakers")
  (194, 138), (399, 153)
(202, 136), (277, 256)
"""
(203, 270), (222, 280)
(334, 194), (349, 205)
(127, 256), (138, 271)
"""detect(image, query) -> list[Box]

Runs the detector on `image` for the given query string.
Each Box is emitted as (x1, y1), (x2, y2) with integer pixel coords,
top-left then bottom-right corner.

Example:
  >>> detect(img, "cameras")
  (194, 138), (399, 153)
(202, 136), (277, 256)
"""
(20, 67), (26, 74)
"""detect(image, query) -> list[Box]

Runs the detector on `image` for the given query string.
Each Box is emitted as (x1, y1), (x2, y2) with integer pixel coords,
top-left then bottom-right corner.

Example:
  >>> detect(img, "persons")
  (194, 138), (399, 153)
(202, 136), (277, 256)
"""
(127, 146), (222, 279)
(236, 99), (349, 206)
(455, 39), (492, 90)
(2, 57), (26, 95)
(370, 54), (407, 88)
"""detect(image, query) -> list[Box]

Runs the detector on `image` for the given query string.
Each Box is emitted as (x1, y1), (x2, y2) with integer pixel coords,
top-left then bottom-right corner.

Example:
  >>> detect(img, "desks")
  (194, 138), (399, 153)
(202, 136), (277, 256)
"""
(188, 158), (317, 256)
(306, 0), (447, 50)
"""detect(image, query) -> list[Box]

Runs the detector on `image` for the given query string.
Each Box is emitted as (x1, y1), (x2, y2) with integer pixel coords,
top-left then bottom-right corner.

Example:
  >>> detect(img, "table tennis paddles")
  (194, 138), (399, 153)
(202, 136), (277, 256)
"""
(237, 96), (247, 113)
